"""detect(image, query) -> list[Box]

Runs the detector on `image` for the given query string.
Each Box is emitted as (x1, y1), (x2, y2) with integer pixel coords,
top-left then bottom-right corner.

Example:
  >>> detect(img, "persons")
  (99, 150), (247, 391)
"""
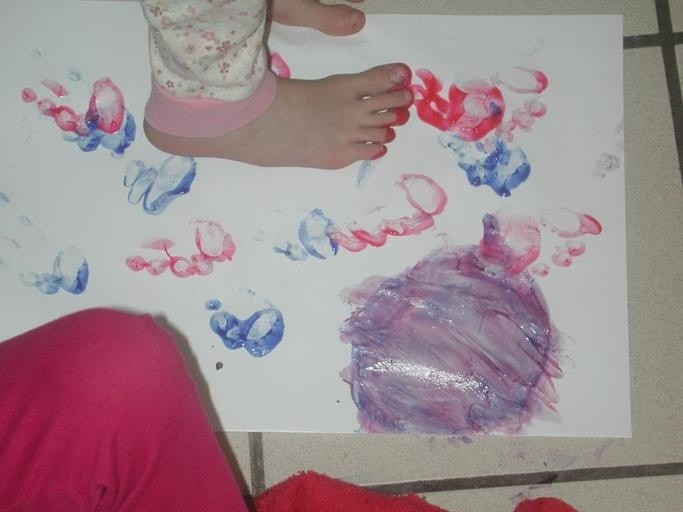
(0, 308), (250, 512)
(138, 0), (413, 171)
